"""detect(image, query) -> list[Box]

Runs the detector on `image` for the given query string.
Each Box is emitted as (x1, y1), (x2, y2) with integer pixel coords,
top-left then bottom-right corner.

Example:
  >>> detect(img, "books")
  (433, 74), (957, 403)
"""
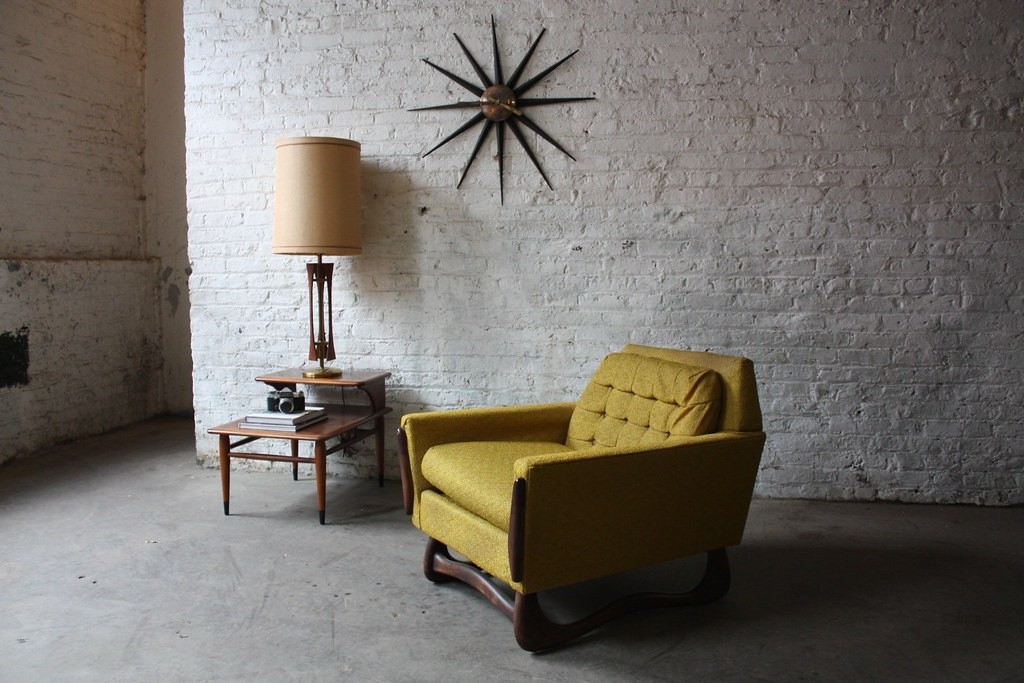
(238, 406), (327, 432)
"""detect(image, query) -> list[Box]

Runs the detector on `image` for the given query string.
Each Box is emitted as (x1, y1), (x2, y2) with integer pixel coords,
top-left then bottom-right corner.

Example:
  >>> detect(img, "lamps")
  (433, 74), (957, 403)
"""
(273, 136), (365, 378)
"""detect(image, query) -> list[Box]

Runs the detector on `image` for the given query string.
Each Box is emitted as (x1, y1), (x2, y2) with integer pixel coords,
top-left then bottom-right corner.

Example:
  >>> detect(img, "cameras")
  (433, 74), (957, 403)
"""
(267, 391), (306, 414)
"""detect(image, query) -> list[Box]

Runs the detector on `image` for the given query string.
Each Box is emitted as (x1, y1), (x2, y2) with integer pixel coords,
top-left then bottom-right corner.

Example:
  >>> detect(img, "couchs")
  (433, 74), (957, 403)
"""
(393, 344), (766, 656)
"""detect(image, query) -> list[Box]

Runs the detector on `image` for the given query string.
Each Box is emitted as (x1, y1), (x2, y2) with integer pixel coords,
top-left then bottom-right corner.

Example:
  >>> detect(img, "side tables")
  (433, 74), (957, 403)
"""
(207, 366), (393, 525)
